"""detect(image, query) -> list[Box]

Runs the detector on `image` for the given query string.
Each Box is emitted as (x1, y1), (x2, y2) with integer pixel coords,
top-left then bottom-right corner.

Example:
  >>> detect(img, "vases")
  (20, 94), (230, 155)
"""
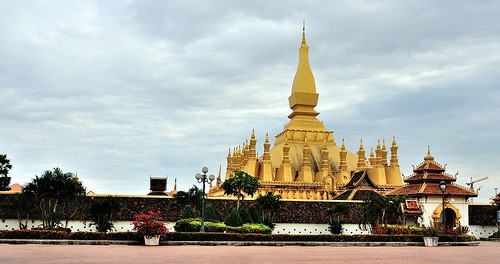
(143, 234), (161, 246)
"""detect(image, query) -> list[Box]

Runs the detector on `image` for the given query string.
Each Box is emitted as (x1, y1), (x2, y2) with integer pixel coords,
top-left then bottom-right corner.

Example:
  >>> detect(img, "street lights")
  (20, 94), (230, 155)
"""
(195, 166), (214, 233)
(439, 181), (448, 225)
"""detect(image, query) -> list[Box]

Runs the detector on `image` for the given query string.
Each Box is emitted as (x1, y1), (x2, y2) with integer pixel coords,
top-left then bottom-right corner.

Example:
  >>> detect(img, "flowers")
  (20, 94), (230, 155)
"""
(130, 208), (168, 238)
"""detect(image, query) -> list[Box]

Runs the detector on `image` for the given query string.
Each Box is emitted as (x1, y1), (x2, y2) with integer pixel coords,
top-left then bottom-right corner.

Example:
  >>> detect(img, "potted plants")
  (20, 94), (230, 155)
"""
(423, 225), (439, 247)
